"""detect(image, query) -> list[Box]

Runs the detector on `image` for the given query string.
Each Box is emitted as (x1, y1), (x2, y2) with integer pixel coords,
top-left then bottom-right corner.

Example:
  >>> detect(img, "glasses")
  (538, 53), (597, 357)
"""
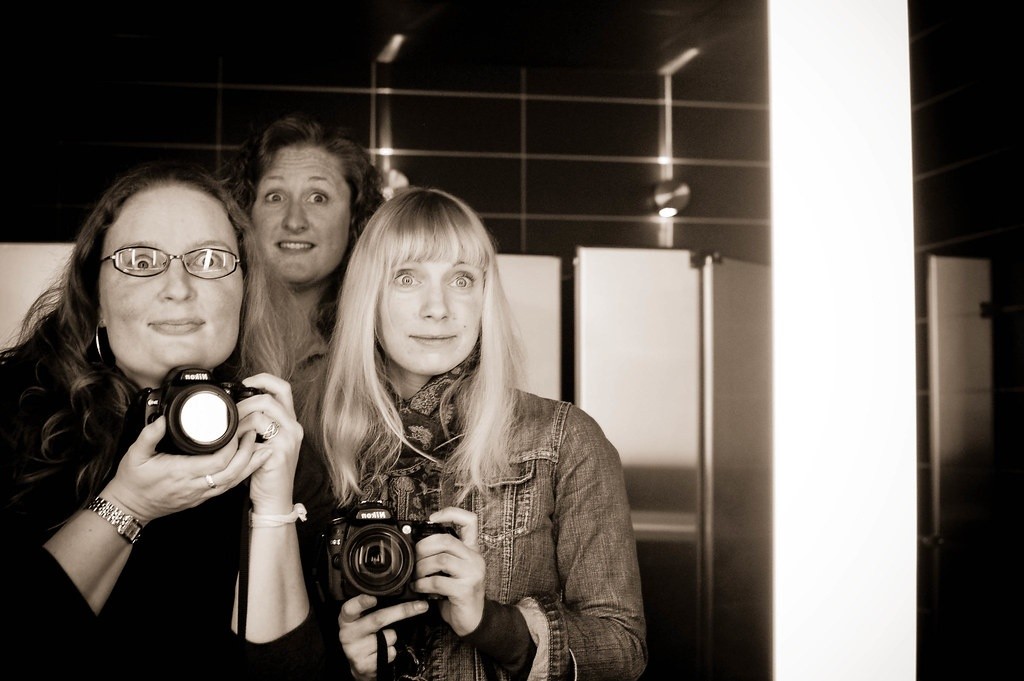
(101, 246), (244, 280)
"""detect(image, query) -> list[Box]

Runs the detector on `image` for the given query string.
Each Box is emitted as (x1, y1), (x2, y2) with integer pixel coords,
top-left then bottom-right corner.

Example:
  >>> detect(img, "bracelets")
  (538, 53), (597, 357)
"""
(248, 502), (308, 528)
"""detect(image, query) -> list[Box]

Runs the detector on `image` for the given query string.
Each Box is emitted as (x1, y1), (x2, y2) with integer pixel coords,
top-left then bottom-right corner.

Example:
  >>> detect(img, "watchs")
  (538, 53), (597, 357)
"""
(81, 496), (146, 546)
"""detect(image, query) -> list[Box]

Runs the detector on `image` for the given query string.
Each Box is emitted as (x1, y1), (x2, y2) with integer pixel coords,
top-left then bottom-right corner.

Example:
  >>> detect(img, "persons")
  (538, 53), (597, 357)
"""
(205, 115), (387, 529)
(0, 161), (328, 681)
(300, 185), (649, 681)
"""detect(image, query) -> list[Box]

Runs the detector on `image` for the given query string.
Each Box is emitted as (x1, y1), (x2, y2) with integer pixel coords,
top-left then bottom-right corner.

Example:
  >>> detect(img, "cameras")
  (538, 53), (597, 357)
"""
(137, 365), (270, 456)
(319, 500), (460, 602)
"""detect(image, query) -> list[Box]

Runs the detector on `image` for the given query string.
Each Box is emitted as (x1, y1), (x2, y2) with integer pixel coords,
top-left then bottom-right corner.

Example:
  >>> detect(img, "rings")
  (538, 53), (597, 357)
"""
(205, 475), (216, 489)
(262, 419), (281, 440)
